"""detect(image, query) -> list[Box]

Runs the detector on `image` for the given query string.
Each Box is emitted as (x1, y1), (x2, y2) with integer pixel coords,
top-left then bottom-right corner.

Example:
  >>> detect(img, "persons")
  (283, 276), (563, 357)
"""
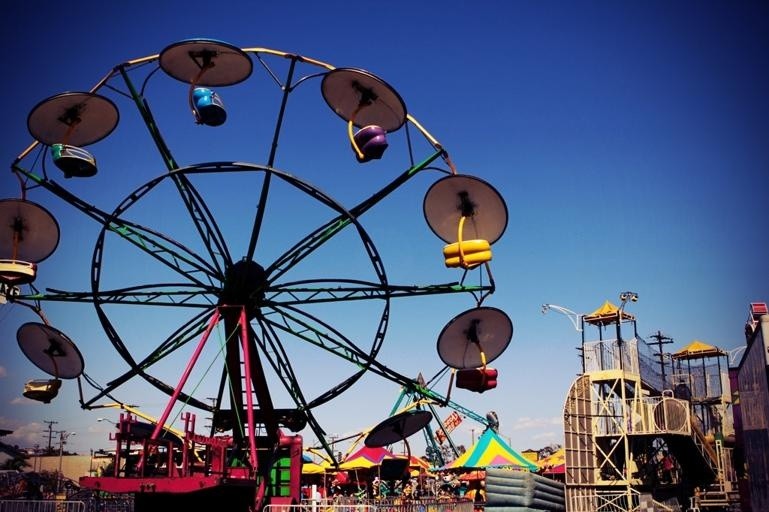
(15, 477), (19, 485)
(676, 380), (692, 407)
(97, 465), (106, 478)
(300, 471), (488, 507)
(1, 475), (8, 489)
(538, 466), (562, 482)
(649, 450), (674, 485)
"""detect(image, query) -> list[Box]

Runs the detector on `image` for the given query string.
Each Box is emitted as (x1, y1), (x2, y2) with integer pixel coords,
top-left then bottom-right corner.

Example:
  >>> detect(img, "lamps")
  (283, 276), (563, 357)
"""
(615, 289), (639, 346)
(540, 303), (586, 332)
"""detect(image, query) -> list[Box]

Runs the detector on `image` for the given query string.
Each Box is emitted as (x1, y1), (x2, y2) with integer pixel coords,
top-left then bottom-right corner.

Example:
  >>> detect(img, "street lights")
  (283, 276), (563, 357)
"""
(57, 431), (77, 493)
(95, 416), (117, 427)
(24, 447), (37, 472)
